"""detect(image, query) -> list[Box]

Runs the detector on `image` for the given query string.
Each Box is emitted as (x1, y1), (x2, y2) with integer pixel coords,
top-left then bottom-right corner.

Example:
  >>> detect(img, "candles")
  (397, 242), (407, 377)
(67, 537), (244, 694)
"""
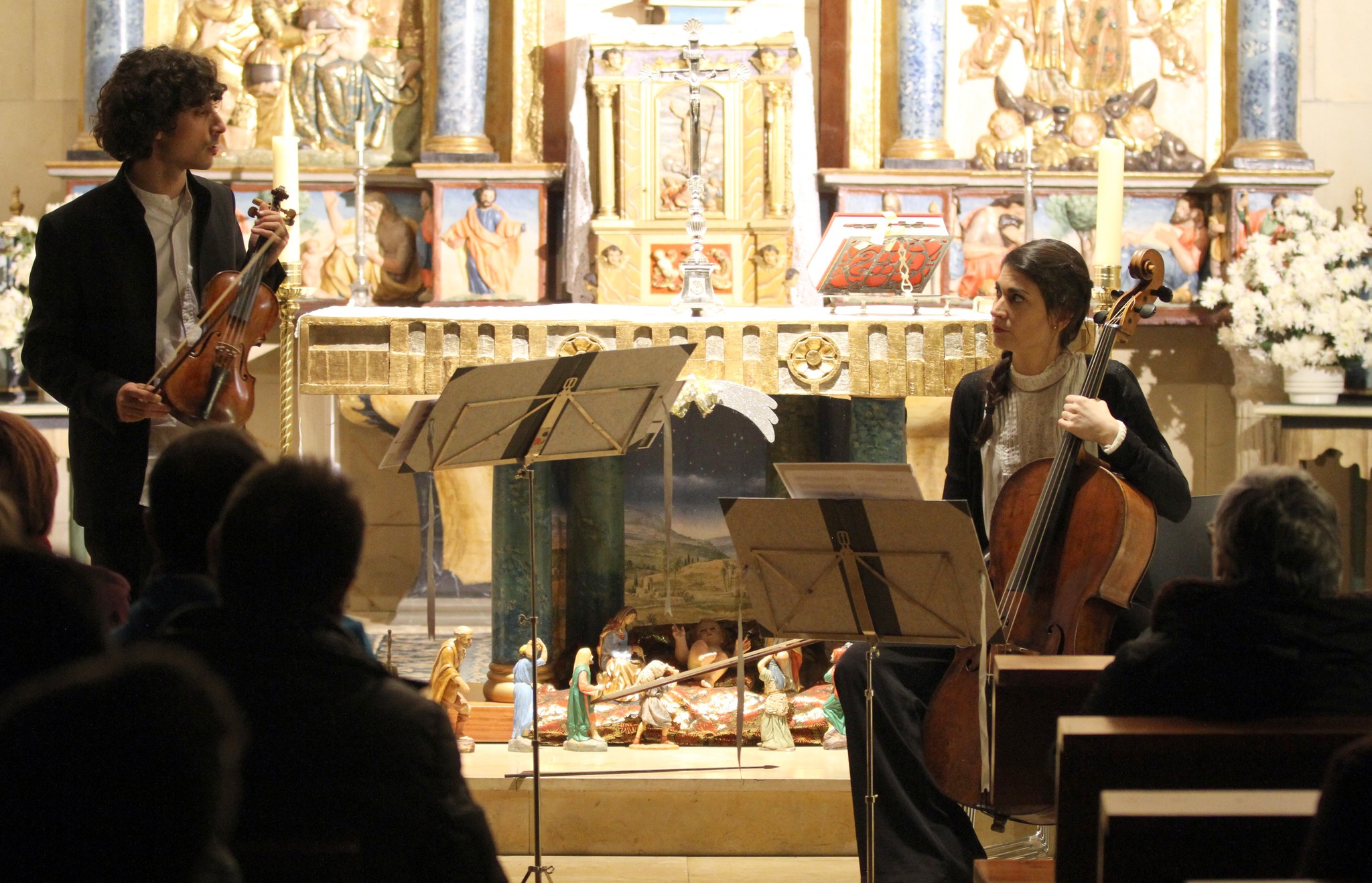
(271, 136), (300, 262)
(1025, 126), (1033, 151)
(1093, 138), (1124, 267)
(354, 121), (365, 151)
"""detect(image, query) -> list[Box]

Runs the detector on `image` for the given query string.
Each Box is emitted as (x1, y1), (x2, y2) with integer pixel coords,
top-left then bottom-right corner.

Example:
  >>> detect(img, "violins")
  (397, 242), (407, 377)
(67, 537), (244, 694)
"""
(158, 184), (296, 431)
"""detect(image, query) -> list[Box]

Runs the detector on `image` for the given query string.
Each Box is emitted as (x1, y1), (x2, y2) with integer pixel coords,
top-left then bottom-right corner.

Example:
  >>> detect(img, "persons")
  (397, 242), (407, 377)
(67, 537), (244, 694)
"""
(1088, 464), (1372, 722)
(20, 47), (290, 595)
(426, 605), (847, 752)
(0, 406), (508, 883)
(833, 239), (1192, 883)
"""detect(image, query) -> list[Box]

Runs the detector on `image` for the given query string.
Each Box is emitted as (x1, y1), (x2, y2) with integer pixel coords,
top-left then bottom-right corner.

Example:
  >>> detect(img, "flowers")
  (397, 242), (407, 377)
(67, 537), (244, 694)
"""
(1199, 194), (1372, 368)
(0, 193), (84, 374)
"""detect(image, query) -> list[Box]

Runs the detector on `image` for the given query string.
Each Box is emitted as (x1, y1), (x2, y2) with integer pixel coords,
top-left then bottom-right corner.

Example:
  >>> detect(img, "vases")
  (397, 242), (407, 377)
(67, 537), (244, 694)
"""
(1283, 363), (1344, 405)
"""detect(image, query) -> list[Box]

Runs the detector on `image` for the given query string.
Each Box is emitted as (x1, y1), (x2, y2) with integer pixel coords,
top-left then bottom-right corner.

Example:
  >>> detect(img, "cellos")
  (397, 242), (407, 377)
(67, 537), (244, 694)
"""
(917, 248), (1175, 823)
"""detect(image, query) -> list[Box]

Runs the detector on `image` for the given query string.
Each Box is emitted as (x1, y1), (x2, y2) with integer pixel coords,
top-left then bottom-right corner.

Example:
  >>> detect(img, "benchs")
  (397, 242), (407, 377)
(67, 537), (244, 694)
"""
(974, 715), (1372, 883)
